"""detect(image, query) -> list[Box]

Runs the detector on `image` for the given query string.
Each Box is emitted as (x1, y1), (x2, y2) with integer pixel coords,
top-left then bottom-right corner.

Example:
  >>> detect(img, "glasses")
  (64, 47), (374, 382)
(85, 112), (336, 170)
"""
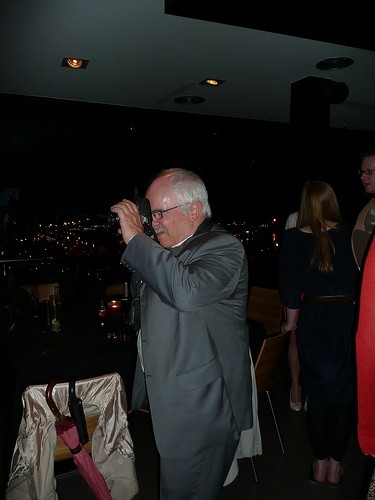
(149, 201), (191, 221)
(358, 169), (375, 175)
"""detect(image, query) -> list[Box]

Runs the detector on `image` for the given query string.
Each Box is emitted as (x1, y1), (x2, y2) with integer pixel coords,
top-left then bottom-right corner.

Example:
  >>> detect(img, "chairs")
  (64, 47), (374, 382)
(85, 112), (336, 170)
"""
(8, 372), (140, 500)
(234, 285), (293, 485)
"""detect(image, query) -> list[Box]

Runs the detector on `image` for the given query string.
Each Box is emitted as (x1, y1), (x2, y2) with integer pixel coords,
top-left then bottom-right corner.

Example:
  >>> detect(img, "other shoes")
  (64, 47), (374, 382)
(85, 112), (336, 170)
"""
(326, 462), (343, 489)
(311, 461), (325, 484)
(289, 386), (301, 411)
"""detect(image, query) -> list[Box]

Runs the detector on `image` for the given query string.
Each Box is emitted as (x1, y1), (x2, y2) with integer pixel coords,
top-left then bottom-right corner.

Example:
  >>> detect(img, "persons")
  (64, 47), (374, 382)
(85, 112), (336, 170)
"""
(271, 181), (358, 489)
(112, 167), (254, 500)
(281, 154), (375, 500)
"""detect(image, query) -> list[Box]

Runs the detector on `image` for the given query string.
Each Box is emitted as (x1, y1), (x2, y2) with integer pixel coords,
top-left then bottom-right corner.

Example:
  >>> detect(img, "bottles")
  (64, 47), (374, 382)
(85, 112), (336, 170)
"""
(108, 214), (148, 228)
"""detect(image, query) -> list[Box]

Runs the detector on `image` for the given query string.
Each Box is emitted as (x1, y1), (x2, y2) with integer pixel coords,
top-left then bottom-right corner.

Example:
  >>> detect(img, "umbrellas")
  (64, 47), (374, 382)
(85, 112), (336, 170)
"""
(46, 380), (111, 500)
